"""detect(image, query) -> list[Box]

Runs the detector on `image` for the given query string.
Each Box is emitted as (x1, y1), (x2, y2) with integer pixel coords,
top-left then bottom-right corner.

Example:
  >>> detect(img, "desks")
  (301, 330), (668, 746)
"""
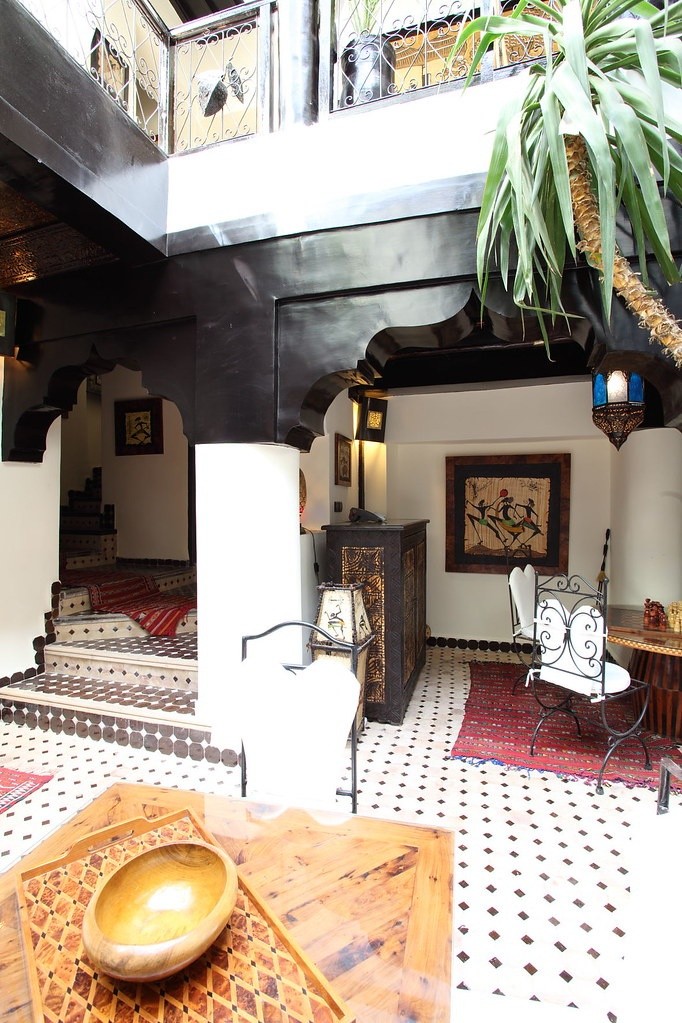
(0, 782), (456, 1023)
(592, 604), (682, 740)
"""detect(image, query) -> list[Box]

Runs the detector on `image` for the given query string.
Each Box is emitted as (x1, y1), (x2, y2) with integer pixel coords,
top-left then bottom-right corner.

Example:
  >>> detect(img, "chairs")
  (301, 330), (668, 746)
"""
(504, 544), (539, 695)
(234, 622), (359, 814)
(526, 570), (652, 796)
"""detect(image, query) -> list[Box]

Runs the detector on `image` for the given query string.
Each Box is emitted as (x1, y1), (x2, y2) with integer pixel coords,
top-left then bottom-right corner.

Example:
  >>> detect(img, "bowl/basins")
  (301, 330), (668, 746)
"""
(81, 839), (239, 984)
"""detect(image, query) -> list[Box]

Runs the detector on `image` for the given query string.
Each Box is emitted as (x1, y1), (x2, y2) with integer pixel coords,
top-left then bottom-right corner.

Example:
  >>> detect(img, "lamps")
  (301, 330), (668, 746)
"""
(17, 345), (38, 371)
(355, 397), (389, 443)
(592, 366), (645, 453)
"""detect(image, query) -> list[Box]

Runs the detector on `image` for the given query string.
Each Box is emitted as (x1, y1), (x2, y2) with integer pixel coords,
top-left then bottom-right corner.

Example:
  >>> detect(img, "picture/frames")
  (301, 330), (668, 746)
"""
(445, 453), (572, 576)
(114, 397), (165, 457)
(334, 432), (353, 487)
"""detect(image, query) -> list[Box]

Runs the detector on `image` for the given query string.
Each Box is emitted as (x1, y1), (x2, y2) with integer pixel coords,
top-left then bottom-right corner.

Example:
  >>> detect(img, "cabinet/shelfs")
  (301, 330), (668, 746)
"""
(321, 519), (431, 726)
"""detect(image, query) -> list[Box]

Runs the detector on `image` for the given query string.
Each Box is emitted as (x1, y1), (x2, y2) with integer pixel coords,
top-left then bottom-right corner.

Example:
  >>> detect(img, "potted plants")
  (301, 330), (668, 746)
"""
(339, 0), (396, 109)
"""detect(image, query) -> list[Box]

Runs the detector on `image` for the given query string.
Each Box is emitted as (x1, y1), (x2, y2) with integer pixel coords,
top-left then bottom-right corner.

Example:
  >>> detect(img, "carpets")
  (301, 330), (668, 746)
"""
(442, 659), (682, 795)
